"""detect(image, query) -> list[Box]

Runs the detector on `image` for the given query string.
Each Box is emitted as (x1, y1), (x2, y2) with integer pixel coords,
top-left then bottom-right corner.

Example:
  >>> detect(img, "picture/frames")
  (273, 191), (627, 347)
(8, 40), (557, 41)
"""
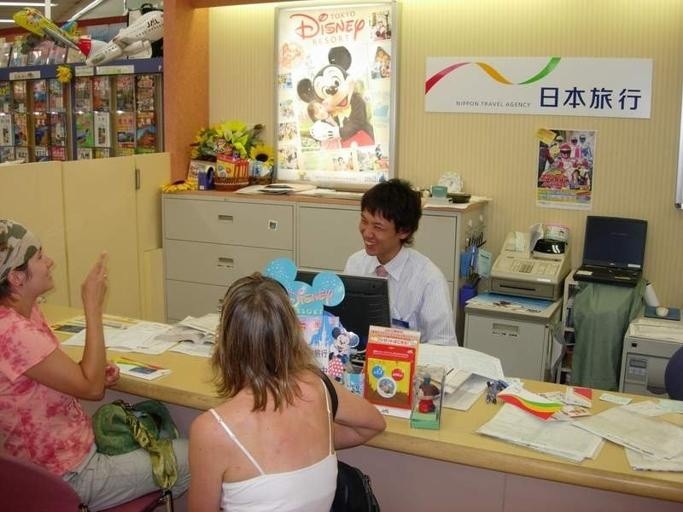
(270, 1), (399, 195)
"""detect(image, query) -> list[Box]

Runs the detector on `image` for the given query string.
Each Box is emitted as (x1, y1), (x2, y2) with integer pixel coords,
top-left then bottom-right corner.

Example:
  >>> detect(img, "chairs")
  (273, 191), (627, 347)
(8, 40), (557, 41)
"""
(663, 347), (682, 400)
(0, 460), (174, 511)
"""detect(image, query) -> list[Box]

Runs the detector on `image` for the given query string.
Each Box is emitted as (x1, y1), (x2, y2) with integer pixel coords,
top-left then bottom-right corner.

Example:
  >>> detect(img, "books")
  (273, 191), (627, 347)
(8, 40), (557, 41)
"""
(0, 37), (158, 163)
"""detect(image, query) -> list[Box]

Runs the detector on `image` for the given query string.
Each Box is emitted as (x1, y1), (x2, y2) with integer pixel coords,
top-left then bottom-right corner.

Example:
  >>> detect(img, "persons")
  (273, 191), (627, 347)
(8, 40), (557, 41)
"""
(538, 134), (591, 192)
(343, 177), (459, 347)
(0, 218), (191, 511)
(186, 270), (389, 511)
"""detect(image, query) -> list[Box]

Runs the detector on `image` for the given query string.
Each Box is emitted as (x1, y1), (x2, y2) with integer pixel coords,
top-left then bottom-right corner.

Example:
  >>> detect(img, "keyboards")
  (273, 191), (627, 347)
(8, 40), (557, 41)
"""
(349, 353), (367, 364)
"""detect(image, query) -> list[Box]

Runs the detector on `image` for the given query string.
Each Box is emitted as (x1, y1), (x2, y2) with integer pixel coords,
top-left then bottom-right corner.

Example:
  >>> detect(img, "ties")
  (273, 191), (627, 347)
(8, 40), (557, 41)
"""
(375, 266), (388, 275)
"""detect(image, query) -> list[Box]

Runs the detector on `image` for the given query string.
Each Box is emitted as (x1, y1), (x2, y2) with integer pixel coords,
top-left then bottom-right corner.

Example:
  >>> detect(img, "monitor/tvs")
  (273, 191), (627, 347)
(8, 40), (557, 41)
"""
(297, 269), (390, 349)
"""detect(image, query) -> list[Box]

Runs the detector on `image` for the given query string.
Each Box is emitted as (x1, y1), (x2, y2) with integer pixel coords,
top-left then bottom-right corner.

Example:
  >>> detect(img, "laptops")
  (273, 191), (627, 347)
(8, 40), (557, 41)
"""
(571, 215), (648, 285)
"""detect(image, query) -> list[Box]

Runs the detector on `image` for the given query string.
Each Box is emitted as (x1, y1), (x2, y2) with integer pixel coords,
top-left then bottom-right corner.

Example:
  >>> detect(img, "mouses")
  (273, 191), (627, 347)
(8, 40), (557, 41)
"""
(655, 306), (668, 316)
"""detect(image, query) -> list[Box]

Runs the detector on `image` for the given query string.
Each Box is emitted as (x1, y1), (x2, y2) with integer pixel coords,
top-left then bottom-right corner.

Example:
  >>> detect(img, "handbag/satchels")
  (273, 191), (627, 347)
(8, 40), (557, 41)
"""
(92, 397), (179, 455)
(330, 462), (380, 512)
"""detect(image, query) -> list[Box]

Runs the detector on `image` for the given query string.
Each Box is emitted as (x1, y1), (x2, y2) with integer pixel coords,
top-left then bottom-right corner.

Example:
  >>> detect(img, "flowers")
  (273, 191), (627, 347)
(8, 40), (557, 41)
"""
(188, 112), (275, 173)
(156, 178), (198, 192)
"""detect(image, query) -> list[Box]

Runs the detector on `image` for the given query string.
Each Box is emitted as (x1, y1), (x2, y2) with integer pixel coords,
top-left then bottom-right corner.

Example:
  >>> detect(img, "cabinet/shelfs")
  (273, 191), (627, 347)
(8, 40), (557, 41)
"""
(555, 265), (647, 393)
(291, 203), (487, 329)
(616, 307), (682, 397)
(157, 193), (292, 329)
(461, 297), (560, 385)
